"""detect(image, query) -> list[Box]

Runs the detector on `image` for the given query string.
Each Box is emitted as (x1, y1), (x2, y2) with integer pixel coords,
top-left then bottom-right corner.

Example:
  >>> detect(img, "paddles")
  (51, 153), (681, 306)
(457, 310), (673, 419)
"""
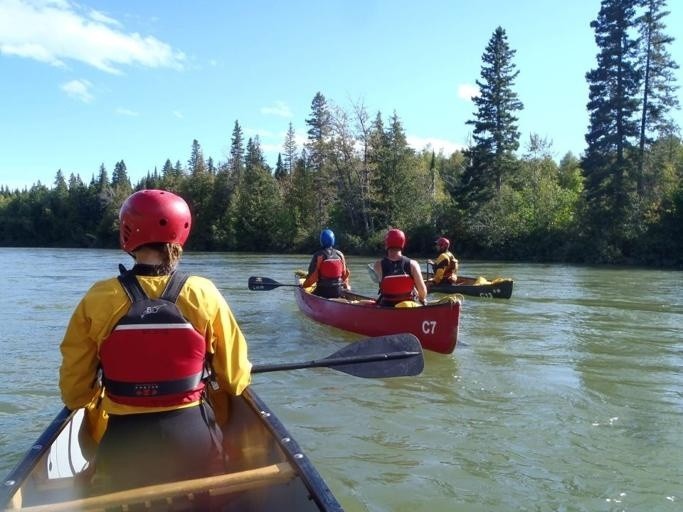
(209, 333), (425, 378)
(248, 276), (317, 292)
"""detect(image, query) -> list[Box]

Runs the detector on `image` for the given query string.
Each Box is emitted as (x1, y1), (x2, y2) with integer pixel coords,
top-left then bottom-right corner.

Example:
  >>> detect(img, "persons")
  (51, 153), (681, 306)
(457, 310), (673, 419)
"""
(426, 238), (459, 287)
(302, 230), (350, 299)
(58, 188), (252, 416)
(370, 228), (428, 306)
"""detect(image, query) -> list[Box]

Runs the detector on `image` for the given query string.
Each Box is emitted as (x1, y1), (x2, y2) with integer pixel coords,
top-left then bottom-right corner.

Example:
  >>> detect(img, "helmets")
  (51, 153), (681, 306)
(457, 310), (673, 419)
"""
(434, 238), (449, 249)
(119, 190), (191, 252)
(319, 229), (335, 247)
(384, 229), (405, 249)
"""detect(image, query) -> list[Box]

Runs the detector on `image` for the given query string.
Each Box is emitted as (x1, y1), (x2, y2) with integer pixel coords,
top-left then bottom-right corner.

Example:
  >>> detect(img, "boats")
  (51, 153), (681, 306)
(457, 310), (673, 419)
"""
(0, 385), (344, 512)
(1, 363), (343, 511)
